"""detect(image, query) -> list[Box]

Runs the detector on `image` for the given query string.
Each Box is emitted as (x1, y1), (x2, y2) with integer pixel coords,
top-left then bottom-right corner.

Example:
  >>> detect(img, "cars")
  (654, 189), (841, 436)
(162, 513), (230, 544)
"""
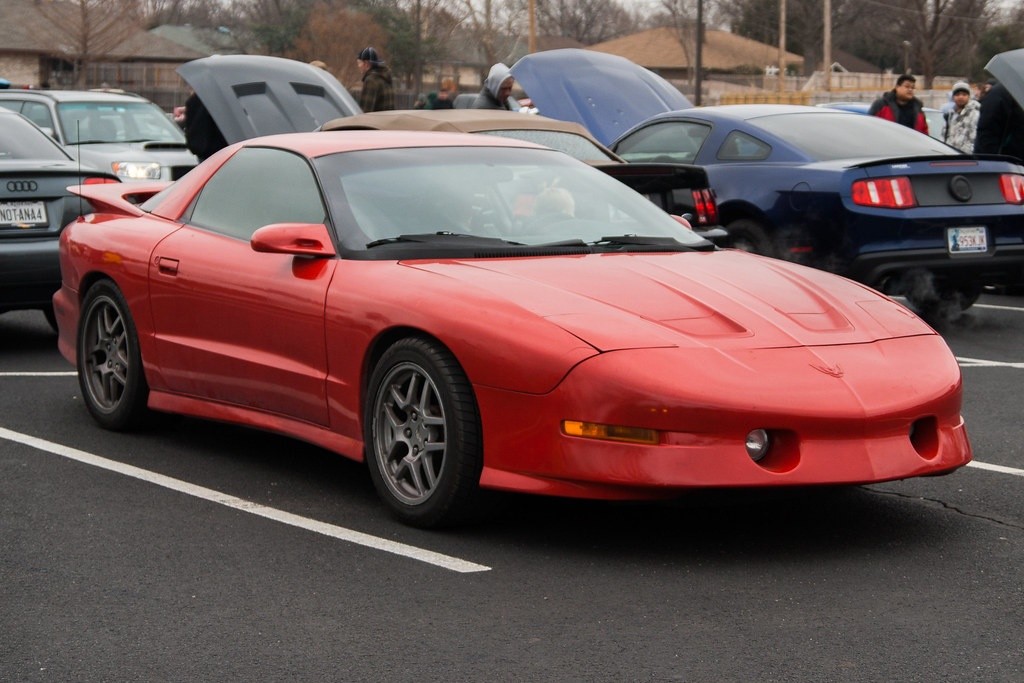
(172, 50), (735, 242)
(969, 46), (1024, 156)
(0, 101), (127, 331)
(507, 47), (1023, 322)
(811, 102), (948, 142)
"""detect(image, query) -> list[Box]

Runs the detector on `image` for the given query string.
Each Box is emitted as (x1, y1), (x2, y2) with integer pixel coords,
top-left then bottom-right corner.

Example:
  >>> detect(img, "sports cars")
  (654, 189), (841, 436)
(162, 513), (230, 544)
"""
(51, 131), (974, 523)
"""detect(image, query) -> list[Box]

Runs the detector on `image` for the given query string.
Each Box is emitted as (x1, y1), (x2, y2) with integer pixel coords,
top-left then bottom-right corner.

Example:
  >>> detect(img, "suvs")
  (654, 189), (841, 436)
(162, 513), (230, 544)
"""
(0, 85), (200, 180)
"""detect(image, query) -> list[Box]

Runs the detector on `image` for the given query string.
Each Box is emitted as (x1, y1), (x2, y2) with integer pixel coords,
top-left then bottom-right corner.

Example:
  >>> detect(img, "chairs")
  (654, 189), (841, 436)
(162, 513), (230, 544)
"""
(88, 120), (117, 139)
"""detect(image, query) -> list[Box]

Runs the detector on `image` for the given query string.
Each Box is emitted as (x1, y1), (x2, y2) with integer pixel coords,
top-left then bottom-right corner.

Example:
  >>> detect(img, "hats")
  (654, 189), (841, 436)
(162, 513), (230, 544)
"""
(985, 79), (996, 86)
(952, 80), (970, 94)
(356, 47), (385, 64)
(309, 59), (327, 70)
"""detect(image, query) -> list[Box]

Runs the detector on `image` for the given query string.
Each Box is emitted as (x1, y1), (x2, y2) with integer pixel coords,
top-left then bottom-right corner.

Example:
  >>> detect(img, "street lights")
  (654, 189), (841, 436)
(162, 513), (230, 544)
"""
(903, 39), (912, 73)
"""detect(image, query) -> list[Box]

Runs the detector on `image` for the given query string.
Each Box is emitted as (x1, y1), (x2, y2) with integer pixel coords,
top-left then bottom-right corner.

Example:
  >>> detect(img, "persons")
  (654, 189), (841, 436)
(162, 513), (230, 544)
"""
(946, 82), (980, 155)
(356, 47), (394, 113)
(184, 92), (228, 161)
(414, 89), (453, 110)
(868, 75), (929, 135)
(473, 63), (514, 111)
(972, 79), (1024, 162)
